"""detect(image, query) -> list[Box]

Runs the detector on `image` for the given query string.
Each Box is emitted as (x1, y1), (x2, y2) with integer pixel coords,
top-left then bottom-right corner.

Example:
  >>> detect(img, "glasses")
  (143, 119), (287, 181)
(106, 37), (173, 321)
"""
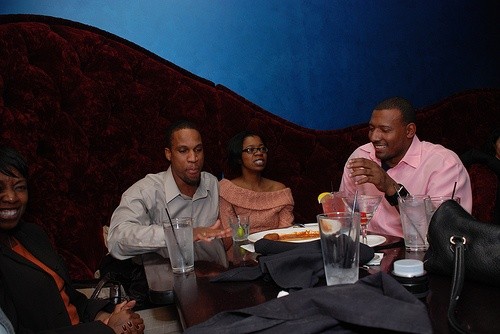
(243, 147), (269, 154)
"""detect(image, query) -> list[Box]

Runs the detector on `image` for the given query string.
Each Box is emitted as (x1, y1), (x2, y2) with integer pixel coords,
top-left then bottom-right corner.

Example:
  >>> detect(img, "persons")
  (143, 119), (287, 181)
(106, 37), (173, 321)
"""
(0, 146), (144, 334)
(107, 122), (219, 261)
(338, 97), (472, 243)
(217, 129), (295, 235)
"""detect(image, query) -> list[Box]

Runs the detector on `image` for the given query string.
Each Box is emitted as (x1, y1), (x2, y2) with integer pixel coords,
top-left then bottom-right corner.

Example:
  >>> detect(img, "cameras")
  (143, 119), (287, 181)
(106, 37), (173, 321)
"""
(109, 286), (121, 313)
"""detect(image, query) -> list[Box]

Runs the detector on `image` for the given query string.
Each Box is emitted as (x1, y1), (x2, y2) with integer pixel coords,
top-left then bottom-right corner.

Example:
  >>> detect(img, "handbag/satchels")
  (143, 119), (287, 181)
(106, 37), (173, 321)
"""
(424, 199), (500, 334)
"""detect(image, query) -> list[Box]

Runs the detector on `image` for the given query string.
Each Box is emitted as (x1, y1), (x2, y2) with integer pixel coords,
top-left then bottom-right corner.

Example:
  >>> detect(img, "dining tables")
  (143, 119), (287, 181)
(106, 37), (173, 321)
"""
(170, 277), (289, 330)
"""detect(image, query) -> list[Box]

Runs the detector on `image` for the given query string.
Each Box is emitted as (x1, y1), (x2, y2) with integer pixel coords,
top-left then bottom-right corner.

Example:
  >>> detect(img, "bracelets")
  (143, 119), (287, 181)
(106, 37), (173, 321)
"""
(386, 184), (409, 206)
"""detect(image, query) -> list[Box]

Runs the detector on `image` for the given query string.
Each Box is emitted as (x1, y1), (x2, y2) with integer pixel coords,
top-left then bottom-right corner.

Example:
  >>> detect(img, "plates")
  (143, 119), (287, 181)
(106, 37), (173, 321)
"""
(246, 227), (339, 243)
(358, 234), (386, 247)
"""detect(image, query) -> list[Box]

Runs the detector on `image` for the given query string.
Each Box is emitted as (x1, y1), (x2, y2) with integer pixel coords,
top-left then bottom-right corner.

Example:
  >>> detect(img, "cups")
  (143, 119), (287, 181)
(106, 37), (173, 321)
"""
(228, 214), (250, 242)
(317, 212), (361, 286)
(161, 217), (195, 273)
(397, 195), (430, 251)
(321, 196), (349, 224)
(424, 196), (460, 226)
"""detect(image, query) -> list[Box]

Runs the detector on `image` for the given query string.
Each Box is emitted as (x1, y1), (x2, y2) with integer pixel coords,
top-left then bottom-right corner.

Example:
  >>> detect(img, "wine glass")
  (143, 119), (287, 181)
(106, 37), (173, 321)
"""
(342, 195), (383, 244)
